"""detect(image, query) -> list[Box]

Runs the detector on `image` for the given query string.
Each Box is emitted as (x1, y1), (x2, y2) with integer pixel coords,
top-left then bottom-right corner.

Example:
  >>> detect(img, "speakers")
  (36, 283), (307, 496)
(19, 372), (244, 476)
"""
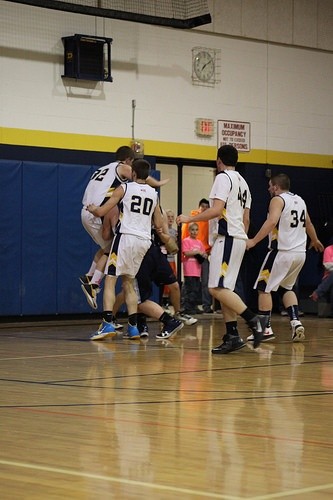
(66, 40), (104, 75)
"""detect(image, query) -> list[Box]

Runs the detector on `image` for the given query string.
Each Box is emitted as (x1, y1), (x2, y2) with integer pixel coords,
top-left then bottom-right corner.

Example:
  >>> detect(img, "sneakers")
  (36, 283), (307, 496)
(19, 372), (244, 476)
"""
(155, 319), (185, 339)
(121, 322), (141, 340)
(211, 332), (246, 354)
(201, 306), (213, 313)
(174, 311), (198, 326)
(137, 324), (149, 338)
(89, 319), (115, 340)
(247, 325), (275, 341)
(247, 314), (271, 349)
(80, 273), (100, 309)
(98, 322), (124, 330)
(290, 319), (305, 343)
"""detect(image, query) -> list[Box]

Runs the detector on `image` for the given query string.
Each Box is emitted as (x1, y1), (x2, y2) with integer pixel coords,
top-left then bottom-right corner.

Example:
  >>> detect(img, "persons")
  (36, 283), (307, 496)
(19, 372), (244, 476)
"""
(108, 203), (199, 329)
(307, 244), (333, 307)
(181, 197), (213, 314)
(101, 201), (185, 339)
(179, 221), (208, 315)
(163, 209), (180, 309)
(86, 159), (165, 341)
(80, 145), (172, 309)
(177, 145), (270, 353)
(244, 172), (325, 343)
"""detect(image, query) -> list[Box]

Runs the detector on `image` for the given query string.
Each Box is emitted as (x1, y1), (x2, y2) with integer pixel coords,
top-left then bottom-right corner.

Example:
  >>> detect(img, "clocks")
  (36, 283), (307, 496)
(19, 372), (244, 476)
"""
(191, 46), (222, 85)
